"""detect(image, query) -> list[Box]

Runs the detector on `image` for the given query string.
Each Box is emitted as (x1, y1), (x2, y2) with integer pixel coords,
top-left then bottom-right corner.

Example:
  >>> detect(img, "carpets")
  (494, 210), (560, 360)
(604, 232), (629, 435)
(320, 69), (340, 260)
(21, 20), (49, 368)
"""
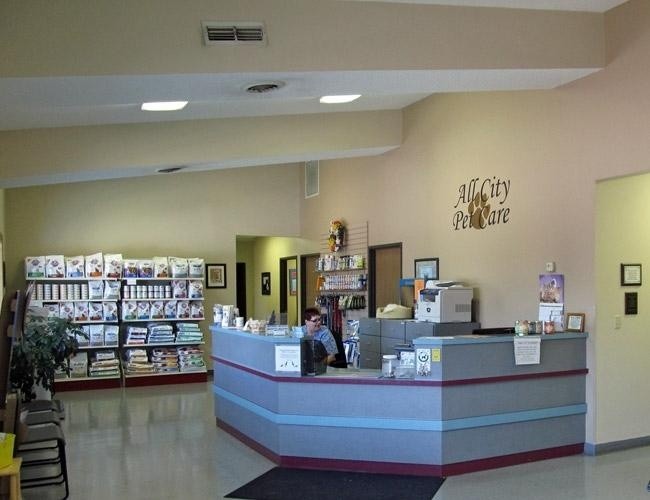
(224, 468), (447, 500)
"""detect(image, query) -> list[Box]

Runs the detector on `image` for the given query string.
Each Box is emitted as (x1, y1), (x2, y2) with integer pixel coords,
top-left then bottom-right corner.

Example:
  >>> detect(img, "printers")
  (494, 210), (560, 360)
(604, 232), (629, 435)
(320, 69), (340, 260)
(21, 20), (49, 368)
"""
(417, 280), (474, 323)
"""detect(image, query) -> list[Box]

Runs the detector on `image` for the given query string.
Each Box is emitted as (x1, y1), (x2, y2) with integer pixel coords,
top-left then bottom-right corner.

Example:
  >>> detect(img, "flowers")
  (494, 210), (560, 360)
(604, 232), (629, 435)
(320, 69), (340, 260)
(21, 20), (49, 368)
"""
(328, 220), (346, 253)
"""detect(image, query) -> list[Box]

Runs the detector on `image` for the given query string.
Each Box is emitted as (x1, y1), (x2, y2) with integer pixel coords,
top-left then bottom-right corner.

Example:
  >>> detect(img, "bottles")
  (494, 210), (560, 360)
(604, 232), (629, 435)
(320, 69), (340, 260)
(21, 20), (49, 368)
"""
(381, 354), (399, 379)
(515, 320), (555, 335)
(316, 254), (363, 272)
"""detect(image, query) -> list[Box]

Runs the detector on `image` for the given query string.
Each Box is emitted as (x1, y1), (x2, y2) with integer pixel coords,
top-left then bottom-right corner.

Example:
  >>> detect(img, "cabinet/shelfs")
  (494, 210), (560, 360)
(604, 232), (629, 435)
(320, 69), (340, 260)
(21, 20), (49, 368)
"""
(355, 318), (482, 369)
(316, 265), (367, 293)
(123, 278), (209, 387)
(25, 276), (124, 392)
(0, 457), (23, 500)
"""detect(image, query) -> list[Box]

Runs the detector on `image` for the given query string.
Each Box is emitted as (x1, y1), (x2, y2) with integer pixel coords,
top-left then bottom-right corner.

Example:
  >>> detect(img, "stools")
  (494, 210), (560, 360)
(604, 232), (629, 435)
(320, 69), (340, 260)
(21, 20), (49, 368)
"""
(18, 398), (66, 464)
(13, 414), (69, 500)
(15, 401), (66, 488)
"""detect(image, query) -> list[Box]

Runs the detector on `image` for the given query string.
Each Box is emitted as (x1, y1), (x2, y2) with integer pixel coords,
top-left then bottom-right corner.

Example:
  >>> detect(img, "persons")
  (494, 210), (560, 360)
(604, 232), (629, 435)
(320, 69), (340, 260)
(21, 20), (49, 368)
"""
(299, 306), (339, 365)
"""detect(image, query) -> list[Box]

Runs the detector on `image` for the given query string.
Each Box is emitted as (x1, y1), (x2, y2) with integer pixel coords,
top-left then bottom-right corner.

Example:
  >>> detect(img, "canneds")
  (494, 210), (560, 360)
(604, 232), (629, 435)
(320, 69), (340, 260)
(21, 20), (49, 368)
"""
(123, 285), (175, 298)
(514, 319), (542, 336)
(544, 321), (554, 334)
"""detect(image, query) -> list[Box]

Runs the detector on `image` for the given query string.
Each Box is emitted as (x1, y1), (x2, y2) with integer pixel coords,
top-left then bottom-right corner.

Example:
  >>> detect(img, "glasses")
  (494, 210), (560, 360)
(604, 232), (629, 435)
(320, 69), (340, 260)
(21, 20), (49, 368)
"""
(309, 318), (321, 322)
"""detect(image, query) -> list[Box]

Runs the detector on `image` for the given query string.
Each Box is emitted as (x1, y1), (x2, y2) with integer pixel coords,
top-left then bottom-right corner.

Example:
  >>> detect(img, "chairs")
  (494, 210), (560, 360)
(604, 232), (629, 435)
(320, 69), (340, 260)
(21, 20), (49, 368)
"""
(329, 332), (348, 367)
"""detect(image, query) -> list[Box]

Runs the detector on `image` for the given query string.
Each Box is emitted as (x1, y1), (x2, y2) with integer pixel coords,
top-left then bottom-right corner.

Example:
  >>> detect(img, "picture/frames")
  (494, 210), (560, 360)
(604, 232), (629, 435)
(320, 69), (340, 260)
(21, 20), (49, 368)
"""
(620, 262), (642, 287)
(262, 272), (272, 295)
(413, 257), (440, 280)
(565, 313), (585, 334)
(289, 269), (297, 296)
(205, 262), (227, 289)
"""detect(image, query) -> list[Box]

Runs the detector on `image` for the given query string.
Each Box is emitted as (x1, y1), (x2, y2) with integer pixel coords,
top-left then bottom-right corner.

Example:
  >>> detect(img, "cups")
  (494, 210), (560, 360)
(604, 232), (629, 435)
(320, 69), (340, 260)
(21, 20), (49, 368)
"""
(233, 317), (244, 327)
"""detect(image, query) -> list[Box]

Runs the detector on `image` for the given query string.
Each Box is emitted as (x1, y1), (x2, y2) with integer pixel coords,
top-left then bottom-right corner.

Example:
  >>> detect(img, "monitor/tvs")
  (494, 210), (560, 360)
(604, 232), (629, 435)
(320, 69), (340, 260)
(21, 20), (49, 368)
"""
(472, 327), (515, 335)
